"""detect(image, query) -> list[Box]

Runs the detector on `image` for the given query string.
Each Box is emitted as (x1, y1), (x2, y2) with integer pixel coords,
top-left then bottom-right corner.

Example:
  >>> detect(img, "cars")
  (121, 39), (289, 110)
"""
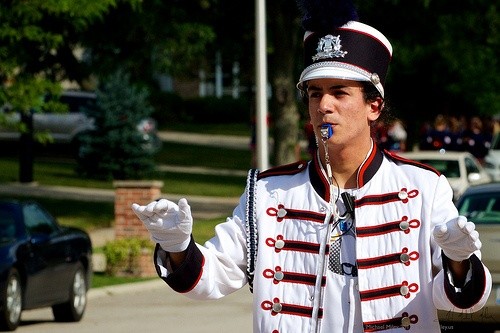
(0, 196), (93, 333)
(395, 150), (493, 205)
(457, 181), (499, 224)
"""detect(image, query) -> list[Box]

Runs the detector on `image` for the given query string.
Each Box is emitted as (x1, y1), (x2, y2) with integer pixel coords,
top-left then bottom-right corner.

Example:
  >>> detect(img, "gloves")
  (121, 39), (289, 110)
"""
(433, 215), (483, 261)
(130, 197), (192, 253)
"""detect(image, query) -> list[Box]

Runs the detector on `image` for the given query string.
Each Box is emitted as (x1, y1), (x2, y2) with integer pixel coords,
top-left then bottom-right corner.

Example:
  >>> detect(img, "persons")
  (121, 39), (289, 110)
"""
(371, 110), (500, 161)
(131, 20), (491, 333)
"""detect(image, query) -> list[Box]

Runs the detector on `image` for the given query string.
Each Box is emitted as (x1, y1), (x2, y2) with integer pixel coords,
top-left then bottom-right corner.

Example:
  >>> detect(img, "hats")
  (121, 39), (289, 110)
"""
(293, 0), (393, 99)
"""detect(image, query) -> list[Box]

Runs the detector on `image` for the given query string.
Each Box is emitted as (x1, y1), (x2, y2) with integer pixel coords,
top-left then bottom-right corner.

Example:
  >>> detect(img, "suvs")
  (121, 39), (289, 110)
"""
(1, 89), (161, 178)
